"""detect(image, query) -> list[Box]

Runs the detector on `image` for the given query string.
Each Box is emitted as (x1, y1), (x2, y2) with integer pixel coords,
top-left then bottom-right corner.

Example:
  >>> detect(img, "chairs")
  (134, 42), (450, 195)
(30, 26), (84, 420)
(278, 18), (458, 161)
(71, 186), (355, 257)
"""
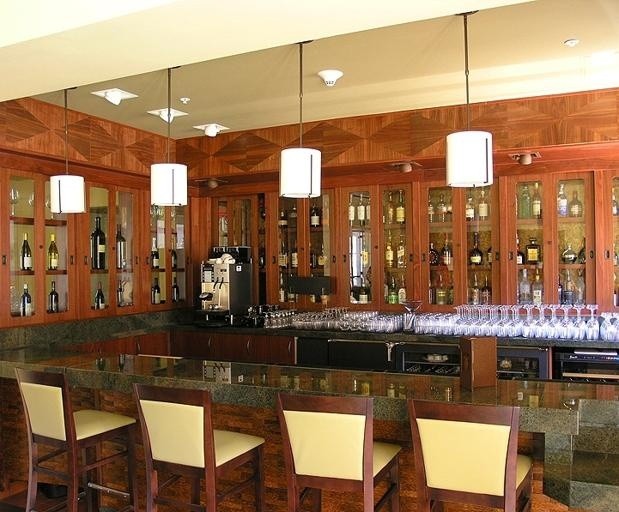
(407, 397), (534, 512)
(132, 382), (266, 512)
(274, 391), (403, 512)
(14, 365), (136, 512)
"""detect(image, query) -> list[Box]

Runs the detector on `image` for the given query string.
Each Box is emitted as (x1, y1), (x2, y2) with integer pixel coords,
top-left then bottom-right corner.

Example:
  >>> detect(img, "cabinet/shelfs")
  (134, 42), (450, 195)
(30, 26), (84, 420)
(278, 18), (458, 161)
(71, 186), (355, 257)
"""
(212, 333), (256, 363)
(209, 191), (305, 311)
(171, 328), (212, 360)
(305, 184), (378, 312)
(142, 183), (189, 310)
(551, 169), (618, 313)
(461, 175), (551, 308)
(256, 334), (296, 365)
(70, 336), (140, 356)
(140, 330), (171, 356)
(77, 181), (142, 319)
(378, 183), (461, 314)
(0, 168), (76, 327)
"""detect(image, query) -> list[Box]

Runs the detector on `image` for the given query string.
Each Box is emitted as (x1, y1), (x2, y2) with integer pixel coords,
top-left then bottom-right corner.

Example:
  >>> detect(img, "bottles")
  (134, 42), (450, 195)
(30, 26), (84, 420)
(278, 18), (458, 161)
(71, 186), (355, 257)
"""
(152, 237), (160, 269)
(116, 223), (127, 270)
(18, 232), (33, 272)
(46, 232), (60, 270)
(97, 356), (105, 371)
(117, 279), (125, 307)
(47, 281), (60, 313)
(90, 216), (107, 270)
(153, 277), (162, 305)
(93, 280), (106, 311)
(170, 239), (178, 270)
(171, 276), (179, 302)
(257, 182), (619, 307)
(20, 283), (32, 318)
(118, 352), (126, 373)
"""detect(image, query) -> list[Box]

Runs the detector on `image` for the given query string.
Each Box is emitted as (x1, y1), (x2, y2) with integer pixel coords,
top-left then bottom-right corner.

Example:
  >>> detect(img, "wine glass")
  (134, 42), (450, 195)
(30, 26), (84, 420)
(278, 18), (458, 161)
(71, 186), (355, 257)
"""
(405, 310), (457, 337)
(45, 195), (54, 220)
(454, 301), (600, 342)
(29, 190), (35, 215)
(10, 189), (20, 217)
(262, 308), (296, 327)
(599, 311), (619, 345)
(323, 309), (406, 334)
(290, 305), (350, 330)
